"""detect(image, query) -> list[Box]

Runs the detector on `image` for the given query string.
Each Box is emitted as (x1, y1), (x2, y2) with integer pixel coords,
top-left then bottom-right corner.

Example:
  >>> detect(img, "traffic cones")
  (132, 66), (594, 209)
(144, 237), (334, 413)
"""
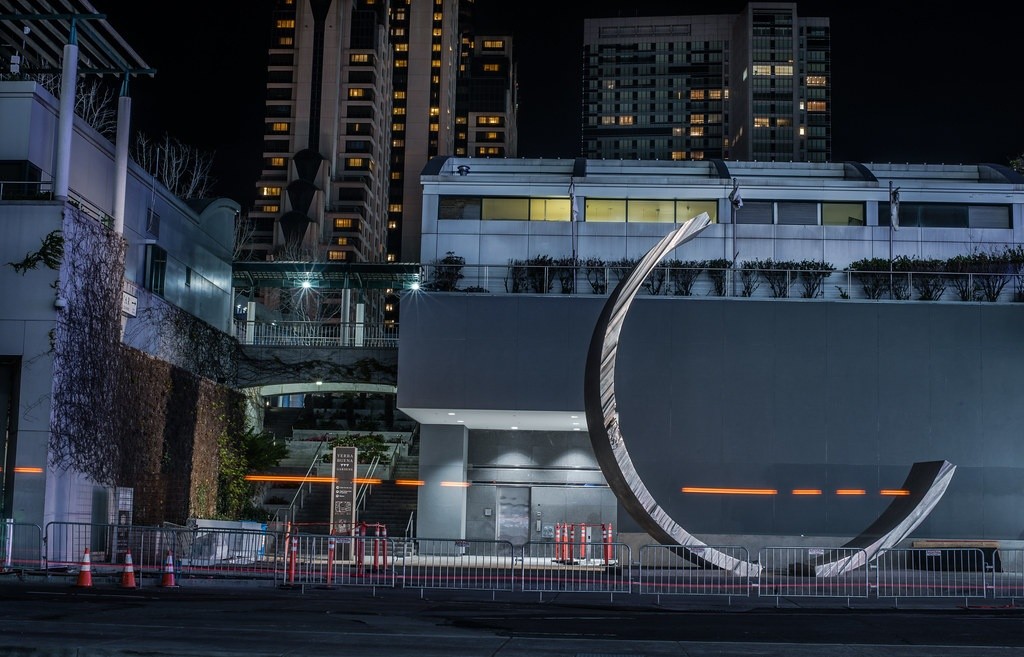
(117, 547), (140, 589)
(156, 551), (180, 589)
(74, 547), (95, 589)
